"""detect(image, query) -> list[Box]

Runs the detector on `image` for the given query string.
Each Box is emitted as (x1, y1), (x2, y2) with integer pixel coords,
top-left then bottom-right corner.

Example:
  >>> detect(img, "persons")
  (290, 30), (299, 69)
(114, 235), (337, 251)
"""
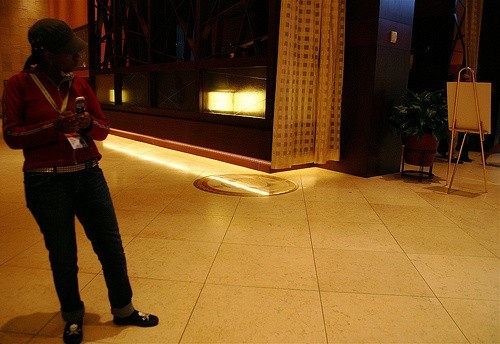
(447, 66), (472, 165)
(0, 18), (161, 344)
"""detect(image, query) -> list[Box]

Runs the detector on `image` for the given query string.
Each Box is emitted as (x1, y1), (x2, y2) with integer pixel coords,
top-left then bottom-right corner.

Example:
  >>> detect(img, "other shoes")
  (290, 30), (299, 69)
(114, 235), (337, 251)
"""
(463, 157), (472, 162)
(452, 158), (463, 164)
(63, 318), (83, 344)
(113, 310), (159, 327)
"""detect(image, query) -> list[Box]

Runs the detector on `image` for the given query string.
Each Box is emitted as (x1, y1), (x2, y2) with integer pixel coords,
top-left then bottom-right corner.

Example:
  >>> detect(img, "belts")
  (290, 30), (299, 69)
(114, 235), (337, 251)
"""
(27, 158), (98, 173)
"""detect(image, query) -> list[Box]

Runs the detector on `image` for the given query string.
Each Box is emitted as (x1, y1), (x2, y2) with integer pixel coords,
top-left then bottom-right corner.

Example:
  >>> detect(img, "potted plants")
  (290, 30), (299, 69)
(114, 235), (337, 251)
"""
(393, 87), (452, 166)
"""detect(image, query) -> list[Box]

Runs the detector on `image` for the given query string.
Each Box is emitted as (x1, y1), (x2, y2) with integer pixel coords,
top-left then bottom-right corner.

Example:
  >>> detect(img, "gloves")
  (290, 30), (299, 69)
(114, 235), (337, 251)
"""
(54, 111), (81, 133)
(73, 112), (90, 131)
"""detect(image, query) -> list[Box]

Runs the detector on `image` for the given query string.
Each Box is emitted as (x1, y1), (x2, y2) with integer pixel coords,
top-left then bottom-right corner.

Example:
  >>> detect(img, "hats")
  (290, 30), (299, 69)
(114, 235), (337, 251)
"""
(27, 17), (89, 54)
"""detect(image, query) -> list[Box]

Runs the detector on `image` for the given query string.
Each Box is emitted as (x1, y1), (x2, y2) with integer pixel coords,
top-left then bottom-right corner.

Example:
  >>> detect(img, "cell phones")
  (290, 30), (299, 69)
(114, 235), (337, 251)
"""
(75, 97), (85, 114)
